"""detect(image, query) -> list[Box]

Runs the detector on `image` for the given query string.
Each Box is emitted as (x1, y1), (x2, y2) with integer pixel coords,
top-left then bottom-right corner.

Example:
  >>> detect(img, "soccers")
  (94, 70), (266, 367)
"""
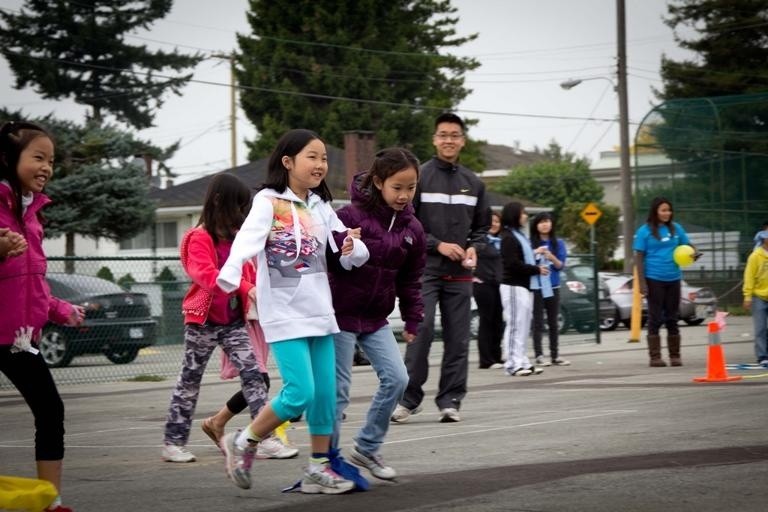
(674, 245), (697, 268)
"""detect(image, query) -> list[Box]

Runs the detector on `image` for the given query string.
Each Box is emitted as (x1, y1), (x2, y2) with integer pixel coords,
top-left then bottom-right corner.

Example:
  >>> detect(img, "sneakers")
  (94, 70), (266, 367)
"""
(489, 354), (569, 375)
(163, 404), (459, 495)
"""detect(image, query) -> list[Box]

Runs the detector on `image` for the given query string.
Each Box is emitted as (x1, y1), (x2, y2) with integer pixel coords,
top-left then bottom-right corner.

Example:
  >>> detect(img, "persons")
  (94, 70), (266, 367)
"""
(216, 130), (371, 495)
(471, 212), (507, 368)
(1, 120), (89, 512)
(527, 209), (566, 366)
(159, 174), (300, 462)
(498, 201), (550, 374)
(741, 225), (768, 367)
(321, 148), (430, 480)
(390, 113), (493, 421)
(202, 256), (271, 448)
(631, 196), (701, 368)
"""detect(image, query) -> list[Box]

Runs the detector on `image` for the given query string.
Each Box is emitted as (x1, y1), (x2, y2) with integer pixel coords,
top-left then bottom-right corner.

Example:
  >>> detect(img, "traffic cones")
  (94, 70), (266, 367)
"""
(693, 322), (744, 385)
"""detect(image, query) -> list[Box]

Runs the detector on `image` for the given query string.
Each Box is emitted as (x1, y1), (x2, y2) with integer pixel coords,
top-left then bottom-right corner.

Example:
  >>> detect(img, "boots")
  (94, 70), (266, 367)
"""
(645, 334), (666, 366)
(667, 335), (682, 366)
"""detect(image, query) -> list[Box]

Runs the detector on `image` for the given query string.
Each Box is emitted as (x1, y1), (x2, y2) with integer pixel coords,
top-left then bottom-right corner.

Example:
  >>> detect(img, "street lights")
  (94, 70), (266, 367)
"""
(559, 74), (633, 279)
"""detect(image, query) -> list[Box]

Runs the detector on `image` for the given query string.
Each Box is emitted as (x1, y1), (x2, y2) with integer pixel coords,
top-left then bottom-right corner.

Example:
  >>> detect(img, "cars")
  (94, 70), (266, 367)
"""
(389, 296), (479, 340)
(538, 261), (618, 333)
(597, 272), (650, 332)
(34, 271), (156, 365)
(678, 281), (719, 326)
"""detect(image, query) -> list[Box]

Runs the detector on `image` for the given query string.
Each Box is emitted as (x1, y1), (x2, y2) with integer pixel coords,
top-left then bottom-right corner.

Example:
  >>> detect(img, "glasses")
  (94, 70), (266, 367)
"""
(435, 134), (462, 140)
